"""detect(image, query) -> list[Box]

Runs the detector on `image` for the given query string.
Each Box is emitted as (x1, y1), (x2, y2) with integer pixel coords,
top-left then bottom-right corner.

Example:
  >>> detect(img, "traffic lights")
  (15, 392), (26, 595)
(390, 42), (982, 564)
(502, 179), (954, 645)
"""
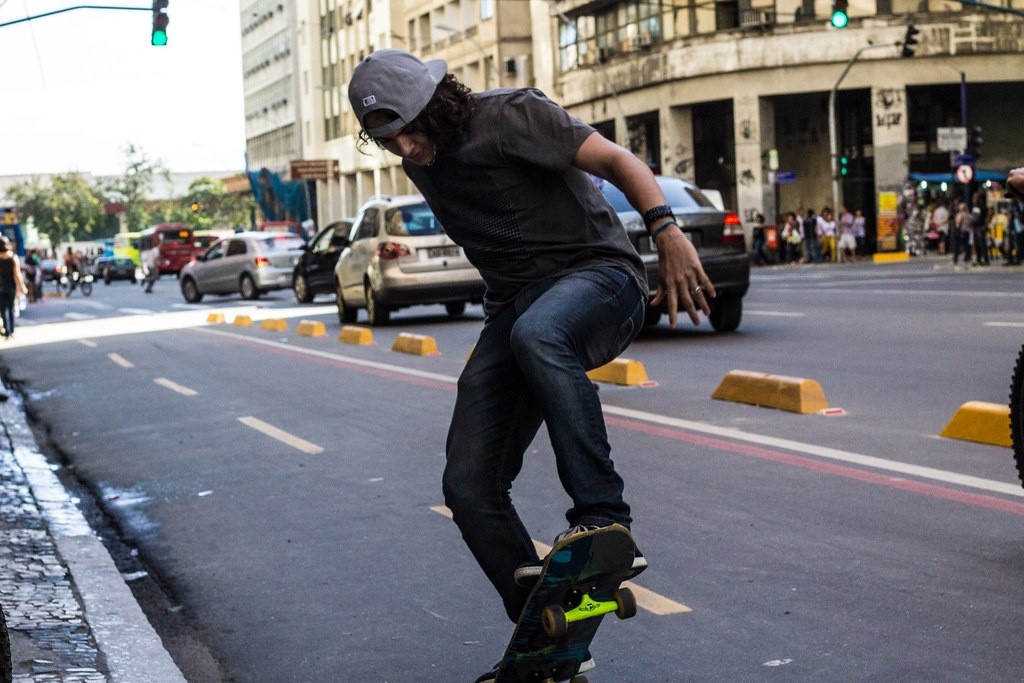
(831, 0), (850, 29)
(192, 198), (200, 216)
(900, 24), (921, 62)
(840, 157), (854, 179)
(152, 0), (171, 47)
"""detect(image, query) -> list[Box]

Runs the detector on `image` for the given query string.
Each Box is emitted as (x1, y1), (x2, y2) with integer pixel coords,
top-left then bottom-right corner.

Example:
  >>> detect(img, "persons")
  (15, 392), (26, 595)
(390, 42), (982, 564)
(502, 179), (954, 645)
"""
(350, 50), (715, 683)
(141, 241), (160, 292)
(751, 206), (865, 264)
(51, 244), (81, 273)
(0, 236), (28, 338)
(25, 249), (42, 302)
(898, 183), (1024, 266)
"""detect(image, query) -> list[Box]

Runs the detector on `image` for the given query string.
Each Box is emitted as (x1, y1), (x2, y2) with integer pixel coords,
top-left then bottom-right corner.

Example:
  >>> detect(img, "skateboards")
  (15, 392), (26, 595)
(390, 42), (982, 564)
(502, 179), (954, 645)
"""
(493, 525), (637, 683)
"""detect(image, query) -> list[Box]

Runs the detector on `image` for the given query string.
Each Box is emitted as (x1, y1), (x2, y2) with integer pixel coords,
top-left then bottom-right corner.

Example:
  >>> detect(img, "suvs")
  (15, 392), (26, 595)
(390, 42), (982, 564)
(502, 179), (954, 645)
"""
(328, 192), (485, 329)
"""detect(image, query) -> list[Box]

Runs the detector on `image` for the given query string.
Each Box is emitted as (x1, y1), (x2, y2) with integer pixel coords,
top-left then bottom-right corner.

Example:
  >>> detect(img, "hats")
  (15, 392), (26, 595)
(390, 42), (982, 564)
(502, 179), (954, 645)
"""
(347, 47), (448, 139)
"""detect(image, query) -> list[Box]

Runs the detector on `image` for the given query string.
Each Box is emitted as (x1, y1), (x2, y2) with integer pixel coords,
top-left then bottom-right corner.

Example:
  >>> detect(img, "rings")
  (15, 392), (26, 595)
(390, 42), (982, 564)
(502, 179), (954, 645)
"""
(691, 285), (701, 296)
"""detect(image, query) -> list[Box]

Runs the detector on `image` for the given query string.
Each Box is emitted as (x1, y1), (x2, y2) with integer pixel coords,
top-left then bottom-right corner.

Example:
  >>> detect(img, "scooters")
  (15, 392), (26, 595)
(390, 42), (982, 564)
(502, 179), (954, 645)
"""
(57, 265), (94, 299)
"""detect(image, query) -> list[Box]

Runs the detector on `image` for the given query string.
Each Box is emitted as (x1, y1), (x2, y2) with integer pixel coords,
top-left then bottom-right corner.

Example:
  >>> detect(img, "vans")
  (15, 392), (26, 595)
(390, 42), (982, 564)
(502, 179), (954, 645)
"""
(256, 221), (306, 240)
(140, 223), (196, 278)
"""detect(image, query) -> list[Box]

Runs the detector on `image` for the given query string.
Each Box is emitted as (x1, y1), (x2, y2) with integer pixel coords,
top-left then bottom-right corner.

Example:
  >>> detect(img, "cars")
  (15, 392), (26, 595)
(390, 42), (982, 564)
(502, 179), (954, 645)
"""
(36, 238), (117, 283)
(103, 257), (138, 285)
(92, 255), (109, 282)
(179, 231), (308, 304)
(595, 176), (753, 332)
(292, 218), (364, 305)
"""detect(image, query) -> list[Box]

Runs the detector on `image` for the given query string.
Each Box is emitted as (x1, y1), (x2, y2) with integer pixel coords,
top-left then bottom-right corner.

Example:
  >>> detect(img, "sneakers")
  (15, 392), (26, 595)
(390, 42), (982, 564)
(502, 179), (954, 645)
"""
(513, 523), (649, 591)
(473, 644), (597, 683)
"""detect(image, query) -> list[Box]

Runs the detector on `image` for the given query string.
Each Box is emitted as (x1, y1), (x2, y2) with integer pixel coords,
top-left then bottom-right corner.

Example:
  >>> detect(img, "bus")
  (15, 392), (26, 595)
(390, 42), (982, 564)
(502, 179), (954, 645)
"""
(0, 208), (34, 273)
(113, 231), (236, 273)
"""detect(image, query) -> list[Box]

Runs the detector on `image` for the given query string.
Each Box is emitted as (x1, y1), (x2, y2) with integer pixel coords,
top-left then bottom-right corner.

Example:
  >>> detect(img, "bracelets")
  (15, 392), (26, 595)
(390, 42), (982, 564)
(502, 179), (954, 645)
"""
(643, 205), (676, 231)
(652, 220), (678, 242)
